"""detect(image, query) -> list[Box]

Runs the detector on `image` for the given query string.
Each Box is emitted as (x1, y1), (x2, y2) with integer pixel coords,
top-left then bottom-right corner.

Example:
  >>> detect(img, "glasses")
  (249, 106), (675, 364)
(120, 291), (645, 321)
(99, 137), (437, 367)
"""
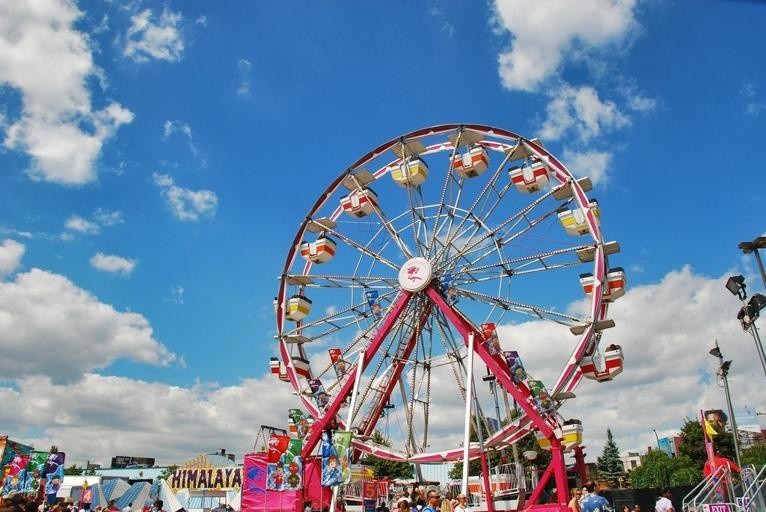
(432, 496), (440, 499)
(576, 492), (582, 495)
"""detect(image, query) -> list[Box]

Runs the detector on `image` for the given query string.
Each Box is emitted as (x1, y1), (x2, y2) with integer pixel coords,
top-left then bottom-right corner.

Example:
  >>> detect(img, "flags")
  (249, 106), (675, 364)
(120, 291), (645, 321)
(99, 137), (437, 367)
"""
(307, 378), (328, 409)
(265, 432), (290, 494)
(281, 434), (304, 492)
(330, 428), (351, 489)
(0, 436), (65, 507)
(288, 408), (310, 437)
(526, 379), (555, 416)
(438, 273), (459, 306)
(481, 321), (502, 356)
(503, 350), (527, 387)
(365, 288), (384, 321)
(320, 429), (344, 489)
(328, 348), (346, 378)
(79, 479), (94, 504)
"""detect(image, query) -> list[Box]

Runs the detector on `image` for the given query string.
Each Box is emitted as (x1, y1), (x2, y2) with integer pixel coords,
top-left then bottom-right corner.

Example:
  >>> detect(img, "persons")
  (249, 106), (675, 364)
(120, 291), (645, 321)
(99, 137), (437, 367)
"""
(654, 486), (676, 512)
(0, 493), (133, 512)
(567, 487), (582, 511)
(608, 344), (617, 351)
(631, 503), (641, 512)
(703, 410), (737, 466)
(303, 500), (312, 512)
(620, 503), (631, 512)
(576, 484), (588, 507)
(577, 479), (613, 511)
(371, 482), (473, 512)
(147, 500), (166, 512)
(703, 438), (743, 505)
